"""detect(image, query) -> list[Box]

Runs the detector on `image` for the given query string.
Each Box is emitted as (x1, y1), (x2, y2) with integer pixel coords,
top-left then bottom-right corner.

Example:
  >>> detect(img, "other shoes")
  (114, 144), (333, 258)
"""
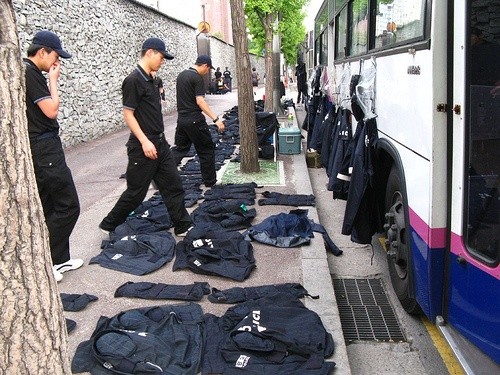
(100, 227), (110, 234)
(201, 187), (211, 197)
(254, 92), (256, 95)
(177, 227), (194, 236)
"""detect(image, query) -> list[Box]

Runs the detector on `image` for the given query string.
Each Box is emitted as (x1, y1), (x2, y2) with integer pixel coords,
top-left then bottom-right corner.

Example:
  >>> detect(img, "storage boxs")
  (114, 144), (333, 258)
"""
(278, 127), (301, 155)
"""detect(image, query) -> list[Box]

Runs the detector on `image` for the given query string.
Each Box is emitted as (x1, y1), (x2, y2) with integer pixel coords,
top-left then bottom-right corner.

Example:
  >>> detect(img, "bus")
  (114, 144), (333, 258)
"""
(313, 0), (500, 364)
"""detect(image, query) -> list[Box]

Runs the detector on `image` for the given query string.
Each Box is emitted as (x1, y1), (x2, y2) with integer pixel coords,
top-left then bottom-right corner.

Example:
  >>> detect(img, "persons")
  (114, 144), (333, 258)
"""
(170, 55), (305, 187)
(23, 30), (84, 280)
(98, 37), (195, 237)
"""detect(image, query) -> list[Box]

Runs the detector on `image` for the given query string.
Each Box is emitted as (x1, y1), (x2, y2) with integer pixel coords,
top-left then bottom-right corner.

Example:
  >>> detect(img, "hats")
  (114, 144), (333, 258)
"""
(33, 31), (71, 58)
(142, 38), (174, 60)
(197, 55), (215, 70)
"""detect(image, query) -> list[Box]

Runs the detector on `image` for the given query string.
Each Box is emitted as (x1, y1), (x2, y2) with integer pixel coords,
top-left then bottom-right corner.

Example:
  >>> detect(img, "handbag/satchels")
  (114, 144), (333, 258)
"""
(257, 75), (260, 80)
(218, 80), (223, 86)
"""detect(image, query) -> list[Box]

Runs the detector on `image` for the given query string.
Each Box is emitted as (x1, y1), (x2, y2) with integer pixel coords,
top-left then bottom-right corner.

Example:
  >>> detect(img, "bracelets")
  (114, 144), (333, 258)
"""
(213, 117), (219, 124)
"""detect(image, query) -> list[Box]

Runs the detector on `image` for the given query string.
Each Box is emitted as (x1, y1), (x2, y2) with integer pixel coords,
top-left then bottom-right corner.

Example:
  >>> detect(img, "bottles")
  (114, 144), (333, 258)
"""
(287, 113), (293, 128)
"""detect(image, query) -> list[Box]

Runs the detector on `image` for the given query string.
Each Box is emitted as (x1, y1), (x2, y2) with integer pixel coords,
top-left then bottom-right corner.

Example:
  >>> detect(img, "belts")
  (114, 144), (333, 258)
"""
(148, 133), (163, 139)
(31, 132), (57, 142)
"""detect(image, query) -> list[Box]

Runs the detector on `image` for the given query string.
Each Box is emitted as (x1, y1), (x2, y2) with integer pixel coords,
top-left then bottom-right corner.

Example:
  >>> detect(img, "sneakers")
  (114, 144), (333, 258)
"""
(53, 271), (63, 282)
(53, 259), (84, 273)
